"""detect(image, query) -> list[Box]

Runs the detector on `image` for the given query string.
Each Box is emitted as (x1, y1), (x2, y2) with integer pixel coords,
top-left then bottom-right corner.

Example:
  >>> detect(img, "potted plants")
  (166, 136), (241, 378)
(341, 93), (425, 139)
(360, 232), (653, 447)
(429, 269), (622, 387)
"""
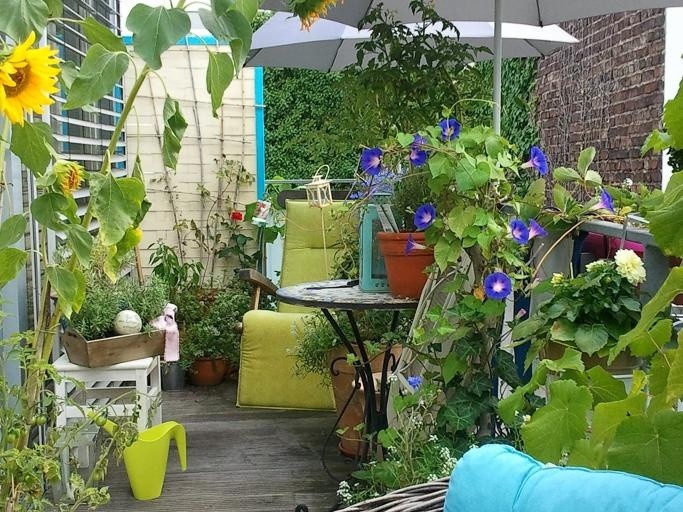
(180, 319), (232, 387)
(62, 265), (171, 368)
(287, 306), (417, 462)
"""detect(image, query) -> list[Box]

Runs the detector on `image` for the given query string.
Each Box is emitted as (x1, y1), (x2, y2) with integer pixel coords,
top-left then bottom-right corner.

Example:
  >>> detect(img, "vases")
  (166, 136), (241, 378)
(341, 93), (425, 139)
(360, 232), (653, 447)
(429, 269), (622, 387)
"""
(537, 335), (643, 374)
(375, 230), (438, 298)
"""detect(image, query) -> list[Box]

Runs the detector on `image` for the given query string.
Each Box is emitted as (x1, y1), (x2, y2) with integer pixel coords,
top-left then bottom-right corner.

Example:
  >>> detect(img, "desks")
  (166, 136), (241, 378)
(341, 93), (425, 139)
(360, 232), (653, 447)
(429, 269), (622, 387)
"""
(50, 351), (161, 468)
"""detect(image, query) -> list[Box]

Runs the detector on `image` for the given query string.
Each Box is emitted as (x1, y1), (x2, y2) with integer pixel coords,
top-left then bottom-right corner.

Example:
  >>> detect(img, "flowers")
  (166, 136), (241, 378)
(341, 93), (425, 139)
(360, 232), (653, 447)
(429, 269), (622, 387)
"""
(511, 244), (647, 375)
(359, 98), (616, 300)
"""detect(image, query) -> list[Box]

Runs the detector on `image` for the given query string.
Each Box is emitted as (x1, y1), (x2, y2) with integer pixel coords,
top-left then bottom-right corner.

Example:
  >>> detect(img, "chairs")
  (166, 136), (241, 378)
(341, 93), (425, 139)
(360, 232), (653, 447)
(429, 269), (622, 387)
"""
(235, 190), (358, 411)
